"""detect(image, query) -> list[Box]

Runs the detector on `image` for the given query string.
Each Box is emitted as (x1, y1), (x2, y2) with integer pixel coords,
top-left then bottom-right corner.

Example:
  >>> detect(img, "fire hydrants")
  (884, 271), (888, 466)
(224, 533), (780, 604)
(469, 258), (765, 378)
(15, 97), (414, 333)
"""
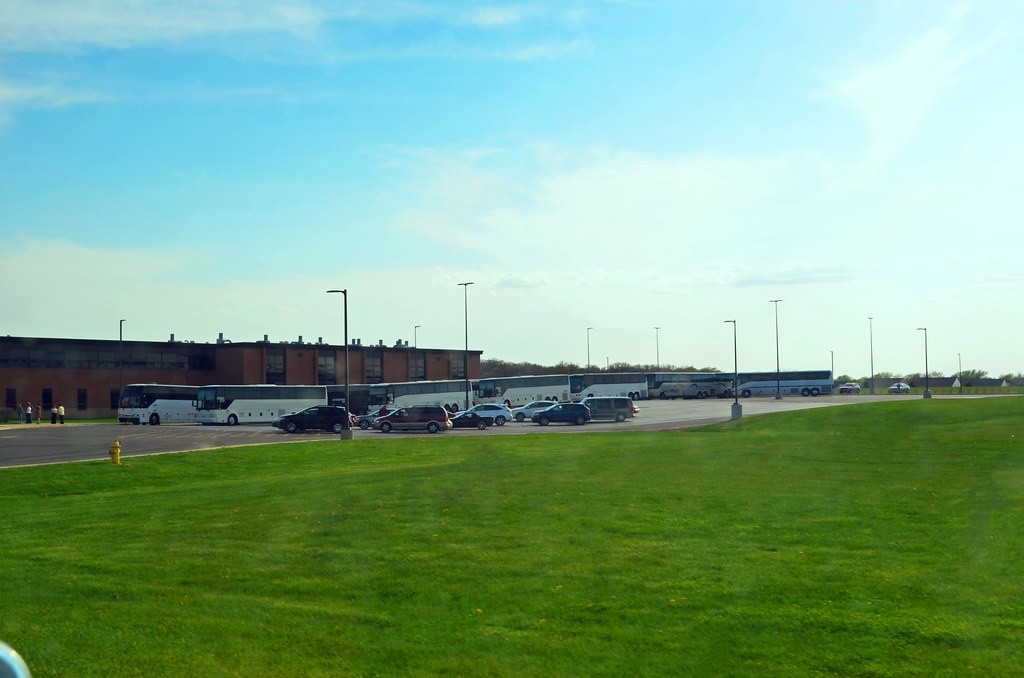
(109, 440), (122, 465)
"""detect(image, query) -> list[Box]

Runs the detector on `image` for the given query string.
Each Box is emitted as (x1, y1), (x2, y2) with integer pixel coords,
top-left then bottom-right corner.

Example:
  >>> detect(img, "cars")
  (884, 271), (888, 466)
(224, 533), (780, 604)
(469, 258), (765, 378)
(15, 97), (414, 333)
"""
(839, 382), (860, 394)
(354, 408), (407, 432)
(888, 382), (911, 393)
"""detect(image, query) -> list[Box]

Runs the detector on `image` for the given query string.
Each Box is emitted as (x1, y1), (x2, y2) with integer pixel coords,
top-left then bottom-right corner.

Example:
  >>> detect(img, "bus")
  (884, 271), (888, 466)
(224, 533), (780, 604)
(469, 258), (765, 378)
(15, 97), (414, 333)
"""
(569, 372), (651, 401)
(116, 383), (200, 426)
(473, 374), (571, 409)
(731, 370), (835, 397)
(365, 380), (474, 413)
(648, 371), (738, 400)
(191, 383), (329, 427)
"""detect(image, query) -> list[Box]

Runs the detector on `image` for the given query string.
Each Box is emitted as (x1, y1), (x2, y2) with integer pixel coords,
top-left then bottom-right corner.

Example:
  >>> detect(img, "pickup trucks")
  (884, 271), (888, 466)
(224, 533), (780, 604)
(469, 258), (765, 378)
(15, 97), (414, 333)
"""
(449, 411), (495, 430)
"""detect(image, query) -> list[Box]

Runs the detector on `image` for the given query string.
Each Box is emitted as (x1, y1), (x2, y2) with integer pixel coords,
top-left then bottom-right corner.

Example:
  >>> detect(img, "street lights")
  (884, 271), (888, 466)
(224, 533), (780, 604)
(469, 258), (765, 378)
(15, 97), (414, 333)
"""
(653, 326), (660, 372)
(769, 299), (783, 399)
(724, 319), (743, 419)
(327, 288), (351, 414)
(868, 316), (876, 395)
(917, 328), (932, 398)
(414, 325), (421, 380)
(118, 319), (126, 388)
(457, 282), (474, 411)
(587, 327), (593, 373)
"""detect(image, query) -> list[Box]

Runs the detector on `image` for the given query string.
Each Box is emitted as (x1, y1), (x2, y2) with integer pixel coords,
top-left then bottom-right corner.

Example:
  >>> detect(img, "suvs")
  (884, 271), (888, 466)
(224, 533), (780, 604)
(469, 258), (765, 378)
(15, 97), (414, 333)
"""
(272, 404), (356, 434)
(511, 401), (560, 422)
(454, 403), (513, 426)
(530, 403), (592, 426)
(371, 404), (454, 433)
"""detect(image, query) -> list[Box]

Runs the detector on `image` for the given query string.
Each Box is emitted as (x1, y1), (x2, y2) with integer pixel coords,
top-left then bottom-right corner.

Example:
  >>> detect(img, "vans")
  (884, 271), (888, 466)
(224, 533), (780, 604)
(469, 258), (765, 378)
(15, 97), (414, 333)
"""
(580, 396), (635, 422)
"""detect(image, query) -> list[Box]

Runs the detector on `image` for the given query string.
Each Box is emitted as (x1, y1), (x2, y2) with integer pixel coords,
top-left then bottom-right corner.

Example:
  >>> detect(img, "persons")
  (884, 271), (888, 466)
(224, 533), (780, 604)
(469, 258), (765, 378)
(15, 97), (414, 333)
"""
(57, 403), (65, 424)
(380, 406), (387, 416)
(16, 403), (23, 421)
(25, 402), (34, 424)
(35, 403), (41, 424)
(51, 404), (58, 424)
